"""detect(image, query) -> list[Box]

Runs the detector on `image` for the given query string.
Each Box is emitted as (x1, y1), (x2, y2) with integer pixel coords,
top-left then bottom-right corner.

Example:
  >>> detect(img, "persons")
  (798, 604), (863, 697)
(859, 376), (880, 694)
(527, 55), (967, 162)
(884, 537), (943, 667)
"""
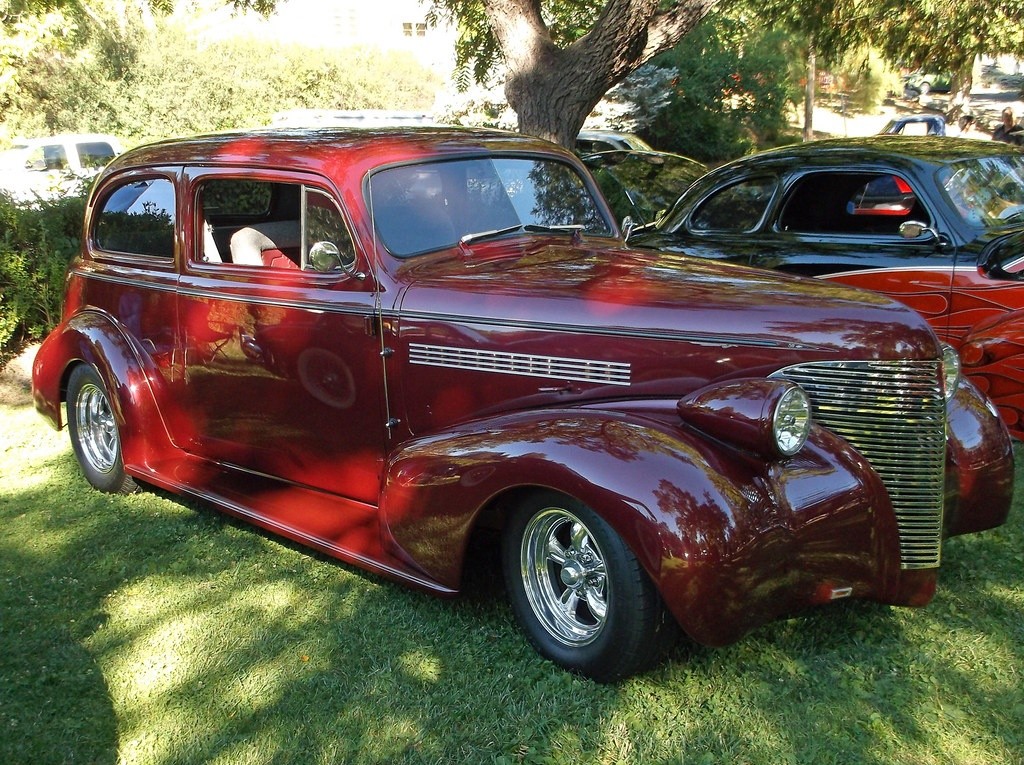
(992, 107), (1024, 147)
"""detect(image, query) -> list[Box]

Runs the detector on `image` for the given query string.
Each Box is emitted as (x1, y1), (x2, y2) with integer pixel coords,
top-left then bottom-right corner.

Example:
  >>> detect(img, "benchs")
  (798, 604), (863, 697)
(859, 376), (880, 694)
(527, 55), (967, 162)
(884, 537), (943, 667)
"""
(105, 225), (242, 265)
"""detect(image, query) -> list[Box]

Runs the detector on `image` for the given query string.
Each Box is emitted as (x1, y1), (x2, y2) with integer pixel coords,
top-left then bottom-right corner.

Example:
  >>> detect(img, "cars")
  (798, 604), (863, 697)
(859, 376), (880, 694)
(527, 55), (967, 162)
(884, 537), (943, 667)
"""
(900, 64), (973, 96)
(1, 132), (123, 211)
(29, 125), (1018, 684)
(574, 112), (1023, 447)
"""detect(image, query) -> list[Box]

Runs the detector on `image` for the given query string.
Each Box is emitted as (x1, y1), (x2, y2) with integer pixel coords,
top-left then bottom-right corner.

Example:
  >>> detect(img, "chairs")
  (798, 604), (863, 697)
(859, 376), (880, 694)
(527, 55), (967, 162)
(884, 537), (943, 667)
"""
(229, 219), (301, 268)
